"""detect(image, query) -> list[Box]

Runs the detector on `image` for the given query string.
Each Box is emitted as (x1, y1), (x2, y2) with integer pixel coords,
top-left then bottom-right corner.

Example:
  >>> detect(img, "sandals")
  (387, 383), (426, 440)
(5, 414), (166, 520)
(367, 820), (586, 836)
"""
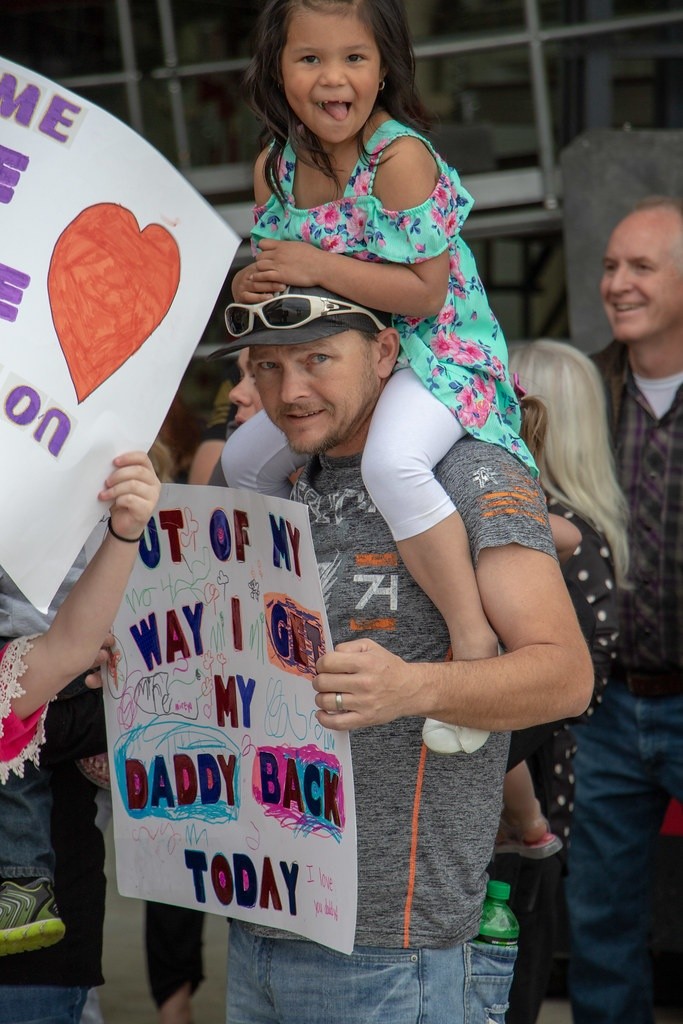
(493, 815), (563, 860)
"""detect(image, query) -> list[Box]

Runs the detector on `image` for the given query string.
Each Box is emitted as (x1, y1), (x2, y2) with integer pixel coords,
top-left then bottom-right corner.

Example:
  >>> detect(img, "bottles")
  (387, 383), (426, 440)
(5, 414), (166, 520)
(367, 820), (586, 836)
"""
(472, 880), (520, 948)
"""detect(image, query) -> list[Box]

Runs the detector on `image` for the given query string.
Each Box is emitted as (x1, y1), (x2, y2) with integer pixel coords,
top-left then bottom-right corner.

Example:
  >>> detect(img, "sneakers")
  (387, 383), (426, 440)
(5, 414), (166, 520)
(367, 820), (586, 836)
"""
(421, 642), (505, 754)
(0, 877), (66, 956)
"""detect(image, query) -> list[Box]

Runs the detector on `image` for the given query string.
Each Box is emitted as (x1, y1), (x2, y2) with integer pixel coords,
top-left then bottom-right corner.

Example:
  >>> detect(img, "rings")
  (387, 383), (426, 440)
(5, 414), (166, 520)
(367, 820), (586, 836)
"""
(336, 692), (343, 711)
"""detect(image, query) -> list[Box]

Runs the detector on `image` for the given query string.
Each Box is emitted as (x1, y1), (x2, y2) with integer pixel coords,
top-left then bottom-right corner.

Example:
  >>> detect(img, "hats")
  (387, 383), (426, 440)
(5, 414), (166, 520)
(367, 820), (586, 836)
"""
(206, 285), (392, 362)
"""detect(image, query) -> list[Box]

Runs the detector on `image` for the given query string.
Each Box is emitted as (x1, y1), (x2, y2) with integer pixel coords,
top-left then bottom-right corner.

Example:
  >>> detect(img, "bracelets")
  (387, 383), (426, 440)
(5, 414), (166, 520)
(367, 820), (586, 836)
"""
(108, 517), (144, 542)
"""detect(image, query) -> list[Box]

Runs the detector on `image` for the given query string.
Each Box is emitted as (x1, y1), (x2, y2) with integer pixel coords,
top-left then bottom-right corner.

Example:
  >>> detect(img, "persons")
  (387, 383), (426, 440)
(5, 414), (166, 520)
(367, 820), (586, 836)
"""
(0, 346), (263, 1024)
(504, 340), (632, 1024)
(0, 451), (161, 784)
(220, 0), (541, 753)
(517, 196), (683, 1024)
(206, 284), (594, 1024)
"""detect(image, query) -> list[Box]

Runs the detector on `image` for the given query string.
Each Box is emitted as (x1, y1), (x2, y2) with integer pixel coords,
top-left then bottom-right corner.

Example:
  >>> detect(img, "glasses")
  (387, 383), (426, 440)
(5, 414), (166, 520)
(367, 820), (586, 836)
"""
(225, 294), (387, 338)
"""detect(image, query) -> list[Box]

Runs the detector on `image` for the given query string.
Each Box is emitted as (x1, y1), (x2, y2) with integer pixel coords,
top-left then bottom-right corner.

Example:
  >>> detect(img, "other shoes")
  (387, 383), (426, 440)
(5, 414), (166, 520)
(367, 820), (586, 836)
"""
(77, 752), (112, 791)
